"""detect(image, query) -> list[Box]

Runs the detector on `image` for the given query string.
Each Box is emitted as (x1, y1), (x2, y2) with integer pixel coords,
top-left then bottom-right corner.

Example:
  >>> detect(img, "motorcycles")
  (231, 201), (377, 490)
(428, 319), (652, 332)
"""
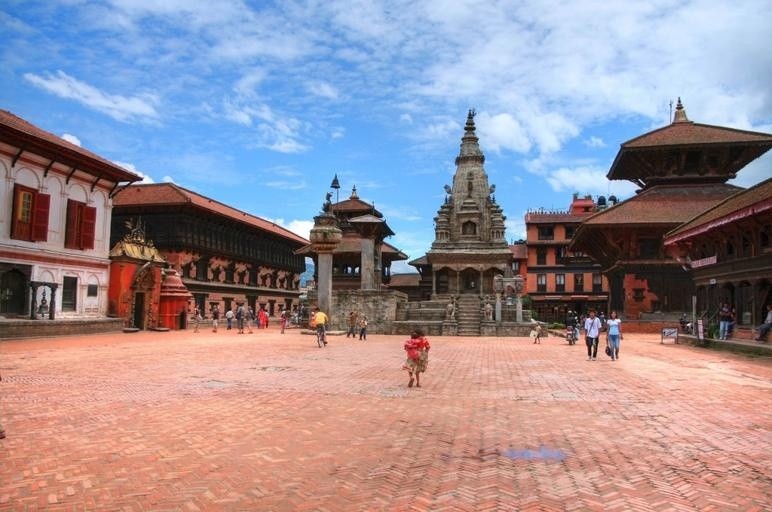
(565, 324), (578, 345)
(679, 311), (693, 333)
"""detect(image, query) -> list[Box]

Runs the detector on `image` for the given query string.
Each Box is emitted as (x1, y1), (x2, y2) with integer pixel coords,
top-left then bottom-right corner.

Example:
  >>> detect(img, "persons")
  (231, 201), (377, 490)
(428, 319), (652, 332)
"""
(606, 310), (624, 360)
(752, 303), (771, 340)
(573, 310), (607, 332)
(565, 310), (580, 340)
(404, 332), (422, 366)
(358, 316), (368, 340)
(312, 307), (329, 344)
(532, 322), (542, 344)
(403, 328), (431, 388)
(193, 303), (302, 334)
(347, 310), (359, 339)
(717, 300), (729, 340)
(726, 301), (736, 336)
(583, 308), (602, 362)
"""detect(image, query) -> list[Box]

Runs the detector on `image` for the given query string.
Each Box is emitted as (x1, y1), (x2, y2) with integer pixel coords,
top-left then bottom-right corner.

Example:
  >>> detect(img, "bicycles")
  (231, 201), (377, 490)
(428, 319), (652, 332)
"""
(317, 322), (329, 348)
